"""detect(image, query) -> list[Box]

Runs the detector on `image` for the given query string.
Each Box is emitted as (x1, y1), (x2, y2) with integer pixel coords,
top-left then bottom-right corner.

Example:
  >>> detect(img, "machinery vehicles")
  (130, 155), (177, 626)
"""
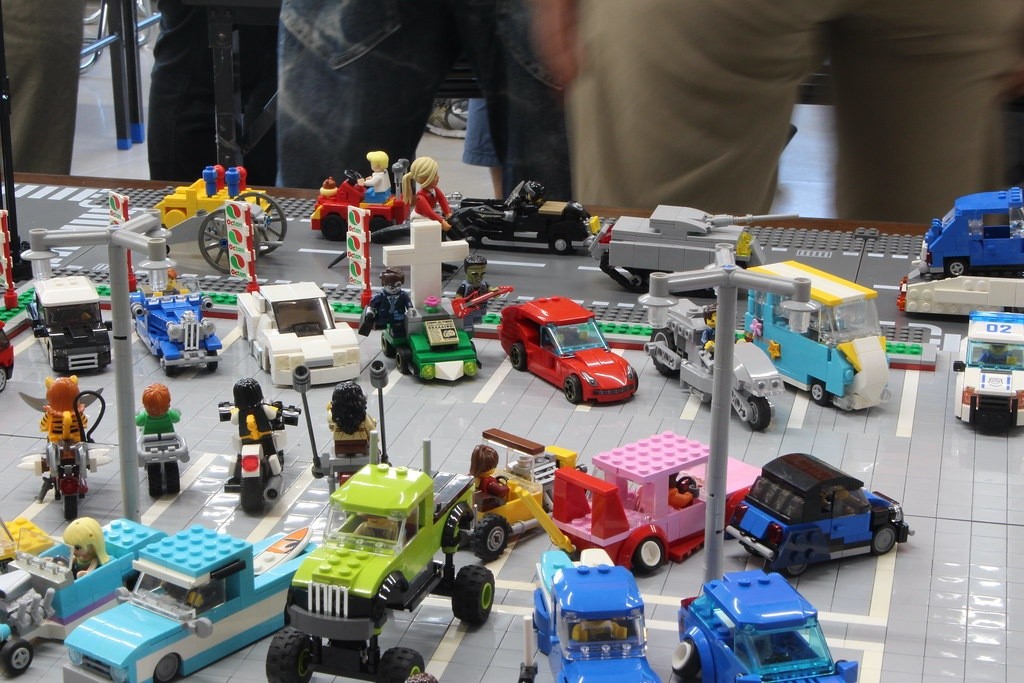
(585, 201), (801, 295)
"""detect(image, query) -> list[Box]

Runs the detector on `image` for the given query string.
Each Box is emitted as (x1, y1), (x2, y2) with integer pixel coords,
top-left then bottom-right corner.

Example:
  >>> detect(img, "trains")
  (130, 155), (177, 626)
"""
(307, 166), (594, 256)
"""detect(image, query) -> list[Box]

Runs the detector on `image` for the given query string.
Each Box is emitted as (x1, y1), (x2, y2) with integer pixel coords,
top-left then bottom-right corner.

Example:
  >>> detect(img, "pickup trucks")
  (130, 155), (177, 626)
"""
(24, 276), (112, 375)
(60, 522), (320, 683)
(532, 546), (665, 683)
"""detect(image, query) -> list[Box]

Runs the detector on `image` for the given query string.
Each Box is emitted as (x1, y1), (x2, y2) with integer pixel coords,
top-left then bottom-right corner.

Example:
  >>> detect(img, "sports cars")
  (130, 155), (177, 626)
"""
(127, 270), (224, 377)
(465, 428), (589, 563)
(0, 518), (170, 679)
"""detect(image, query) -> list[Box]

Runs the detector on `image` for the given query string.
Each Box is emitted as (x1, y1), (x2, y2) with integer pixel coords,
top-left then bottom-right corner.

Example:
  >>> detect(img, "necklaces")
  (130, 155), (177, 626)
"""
(230, 378), (283, 456)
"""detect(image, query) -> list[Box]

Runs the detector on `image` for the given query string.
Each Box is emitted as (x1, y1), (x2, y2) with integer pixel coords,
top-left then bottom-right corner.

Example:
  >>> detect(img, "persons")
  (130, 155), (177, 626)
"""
(366, 266), (418, 330)
(357, 152), (392, 203)
(327, 381), (377, 458)
(636, 472), (700, 516)
(977, 344), (1016, 365)
(470, 446), (518, 498)
(154, 269), (189, 297)
(41, 375), (88, 442)
(136, 383), (180, 435)
(0, 0), (85, 173)
(162, 582), (216, 612)
(701, 304), (746, 361)
(572, 618), (636, 641)
(456, 254), (502, 324)
(45, 518), (110, 580)
(148, 0), (1024, 223)
(354, 515), (407, 541)
(401, 157), (454, 231)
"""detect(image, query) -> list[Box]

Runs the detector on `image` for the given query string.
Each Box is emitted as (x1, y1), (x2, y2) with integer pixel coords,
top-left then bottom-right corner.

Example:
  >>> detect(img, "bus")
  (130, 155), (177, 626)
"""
(741, 258), (893, 413)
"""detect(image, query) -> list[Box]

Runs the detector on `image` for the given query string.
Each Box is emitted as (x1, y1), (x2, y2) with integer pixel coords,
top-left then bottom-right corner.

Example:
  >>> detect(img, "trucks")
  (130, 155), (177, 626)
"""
(909, 185), (1024, 281)
(513, 426), (764, 577)
(264, 428), (497, 683)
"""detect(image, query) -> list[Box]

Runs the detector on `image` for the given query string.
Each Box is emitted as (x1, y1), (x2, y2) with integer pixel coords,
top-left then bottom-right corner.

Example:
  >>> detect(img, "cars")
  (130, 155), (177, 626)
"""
(669, 567), (861, 683)
(0, 320), (15, 393)
(952, 310), (1024, 434)
(498, 294), (640, 405)
(236, 278), (364, 389)
(0, 518), (57, 574)
(644, 297), (787, 432)
(725, 452), (915, 578)
(371, 218), (484, 385)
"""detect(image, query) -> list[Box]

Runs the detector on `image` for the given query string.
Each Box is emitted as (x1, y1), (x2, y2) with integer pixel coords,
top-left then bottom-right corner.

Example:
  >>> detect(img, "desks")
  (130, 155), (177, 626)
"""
(0, 171), (1024, 683)
